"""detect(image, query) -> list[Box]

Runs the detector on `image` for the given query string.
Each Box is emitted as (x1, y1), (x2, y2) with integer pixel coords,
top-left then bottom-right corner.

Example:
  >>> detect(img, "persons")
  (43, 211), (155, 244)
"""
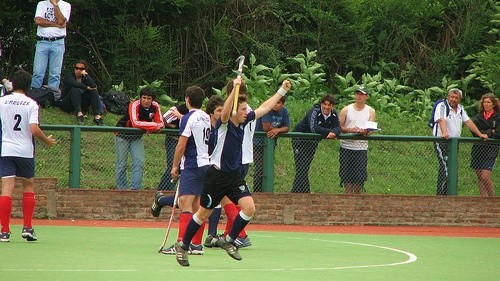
(0, 70), (55, 241)
(339, 86), (376, 195)
(58, 60), (102, 124)
(219, 81), (256, 247)
(252, 95), (290, 192)
(470, 93), (500, 196)
(115, 87), (165, 190)
(158, 105), (189, 190)
(176, 79), (291, 265)
(162, 86), (210, 254)
(292, 94), (342, 193)
(151, 95), (222, 247)
(433, 89), (488, 195)
(31, 0), (71, 106)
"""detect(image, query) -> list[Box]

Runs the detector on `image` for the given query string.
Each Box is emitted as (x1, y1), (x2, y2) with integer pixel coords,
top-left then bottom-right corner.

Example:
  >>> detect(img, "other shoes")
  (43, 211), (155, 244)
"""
(77, 115), (85, 125)
(92, 116), (104, 126)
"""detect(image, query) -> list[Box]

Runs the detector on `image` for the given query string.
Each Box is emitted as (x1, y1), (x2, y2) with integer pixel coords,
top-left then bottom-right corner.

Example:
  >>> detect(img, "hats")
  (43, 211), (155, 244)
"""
(354, 85), (370, 95)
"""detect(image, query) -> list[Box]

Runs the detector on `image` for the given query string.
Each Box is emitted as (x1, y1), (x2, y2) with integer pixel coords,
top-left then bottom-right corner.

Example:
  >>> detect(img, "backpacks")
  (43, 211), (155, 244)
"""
(427, 98), (450, 128)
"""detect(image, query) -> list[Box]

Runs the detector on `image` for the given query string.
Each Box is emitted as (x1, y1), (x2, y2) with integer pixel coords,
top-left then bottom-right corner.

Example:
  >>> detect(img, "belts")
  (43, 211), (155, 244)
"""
(37, 35), (64, 41)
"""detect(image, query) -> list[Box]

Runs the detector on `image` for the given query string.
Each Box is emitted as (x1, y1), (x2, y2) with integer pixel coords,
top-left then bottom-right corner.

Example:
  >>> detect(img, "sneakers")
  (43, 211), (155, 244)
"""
(21, 227), (38, 241)
(202, 230), (252, 261)
(187, 243), (204, 255)
(0, 230), (12, 242)
(151, 191), (163, 217)
(160, 244), (178, 255)
(174, 242), (191, 267)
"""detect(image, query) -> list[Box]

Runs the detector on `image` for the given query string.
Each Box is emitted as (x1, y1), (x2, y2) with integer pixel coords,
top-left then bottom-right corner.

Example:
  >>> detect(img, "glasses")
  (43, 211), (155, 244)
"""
(74, 66), (86, 70)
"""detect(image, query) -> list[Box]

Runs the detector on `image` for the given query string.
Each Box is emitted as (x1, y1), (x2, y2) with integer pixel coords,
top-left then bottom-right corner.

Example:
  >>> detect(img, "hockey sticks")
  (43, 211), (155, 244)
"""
(232, 56), (245, 116)
(158, 177), (180, 253)
